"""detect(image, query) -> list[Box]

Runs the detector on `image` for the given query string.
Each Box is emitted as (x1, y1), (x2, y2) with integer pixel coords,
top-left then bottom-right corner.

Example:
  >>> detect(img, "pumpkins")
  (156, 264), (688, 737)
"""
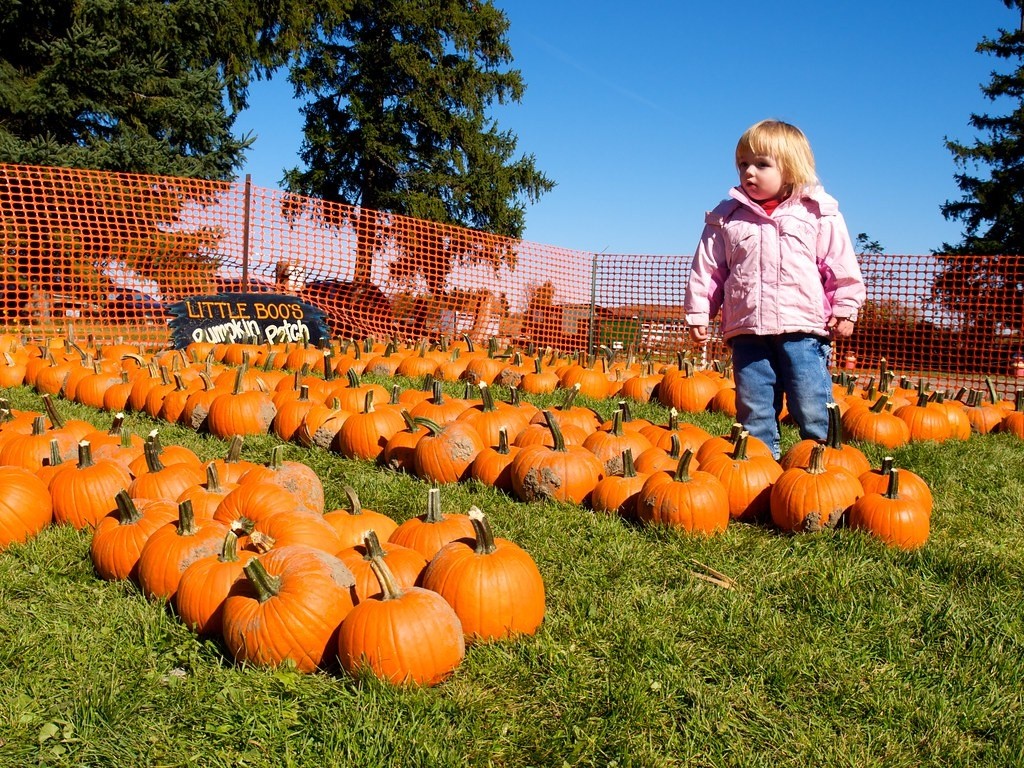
(182, 330), (1024, 447)
(0, 337), (934, 688)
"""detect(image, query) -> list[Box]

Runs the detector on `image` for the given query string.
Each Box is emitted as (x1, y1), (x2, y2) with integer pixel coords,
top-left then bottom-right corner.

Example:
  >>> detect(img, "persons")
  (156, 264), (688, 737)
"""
(684, 121), (868, 460)
